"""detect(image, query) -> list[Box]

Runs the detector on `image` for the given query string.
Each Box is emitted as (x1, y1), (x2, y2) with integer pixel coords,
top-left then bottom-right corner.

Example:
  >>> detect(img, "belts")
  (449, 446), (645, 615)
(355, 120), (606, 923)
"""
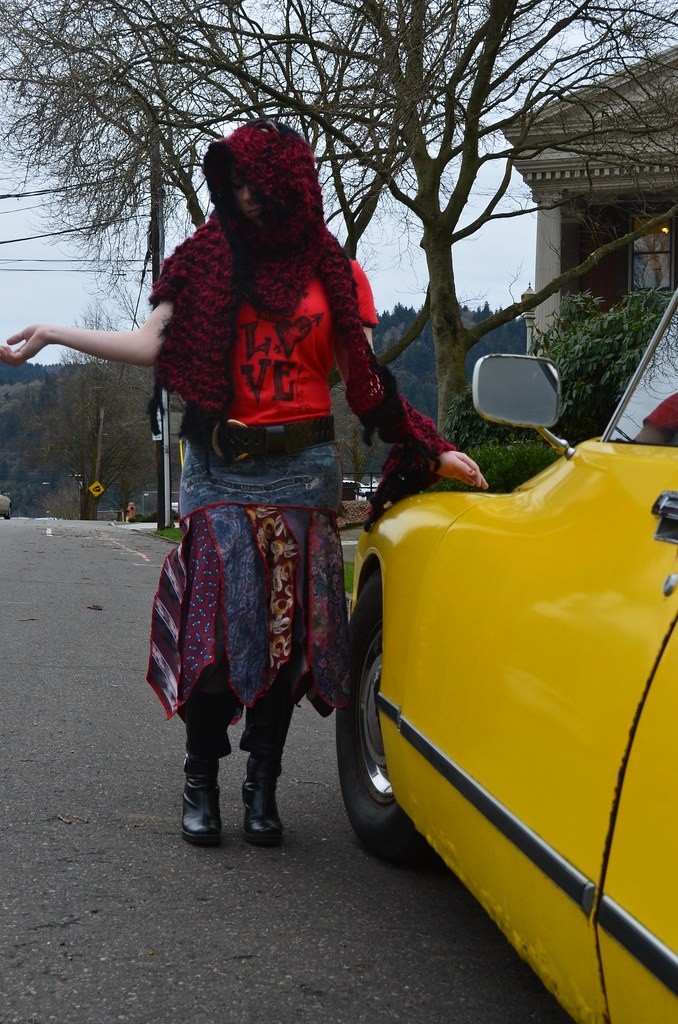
(211, 414), (335, 463)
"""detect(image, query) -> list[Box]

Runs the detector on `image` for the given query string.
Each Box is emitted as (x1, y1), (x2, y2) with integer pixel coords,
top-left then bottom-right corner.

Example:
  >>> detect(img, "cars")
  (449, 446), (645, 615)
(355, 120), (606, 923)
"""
(171, 502), (179, 514)
(0, 489), (12, 520)
(335, 284), (678, 1024)
(343, 476), (380, 502)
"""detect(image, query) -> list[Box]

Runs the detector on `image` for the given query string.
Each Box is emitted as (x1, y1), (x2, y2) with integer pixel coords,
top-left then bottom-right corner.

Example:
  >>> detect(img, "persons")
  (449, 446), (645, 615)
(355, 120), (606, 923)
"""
(634, 390), (678, 445)
(0, 120), (490, 843)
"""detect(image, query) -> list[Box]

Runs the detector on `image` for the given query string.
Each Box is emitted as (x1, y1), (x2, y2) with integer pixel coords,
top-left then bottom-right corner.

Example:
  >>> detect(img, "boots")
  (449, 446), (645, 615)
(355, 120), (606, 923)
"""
(243, 754), (282, 846)
(182, 754), (222, 846)
